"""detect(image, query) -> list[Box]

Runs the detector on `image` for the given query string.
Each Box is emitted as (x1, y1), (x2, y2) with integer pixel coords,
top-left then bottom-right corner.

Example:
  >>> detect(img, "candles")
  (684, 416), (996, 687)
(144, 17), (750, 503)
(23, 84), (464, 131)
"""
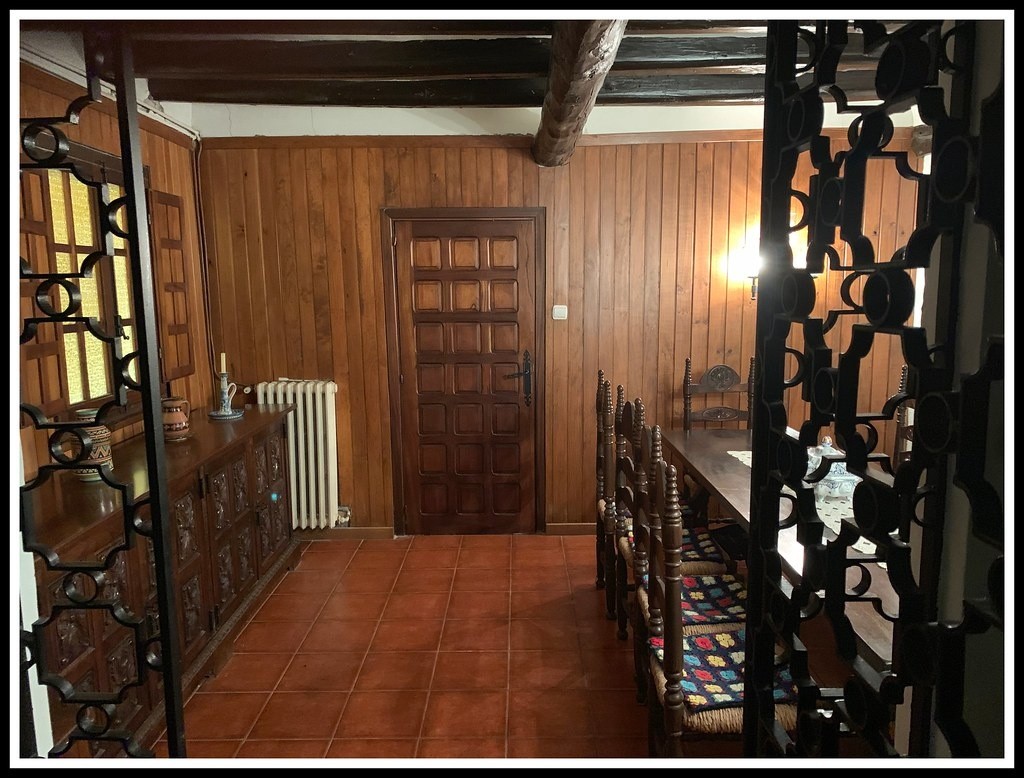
(221, 353), (226, 373)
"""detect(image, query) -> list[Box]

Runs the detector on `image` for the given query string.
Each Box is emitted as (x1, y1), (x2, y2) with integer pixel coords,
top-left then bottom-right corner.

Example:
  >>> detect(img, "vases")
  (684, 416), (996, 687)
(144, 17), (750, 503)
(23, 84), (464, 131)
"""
(70, 408), (114, 482)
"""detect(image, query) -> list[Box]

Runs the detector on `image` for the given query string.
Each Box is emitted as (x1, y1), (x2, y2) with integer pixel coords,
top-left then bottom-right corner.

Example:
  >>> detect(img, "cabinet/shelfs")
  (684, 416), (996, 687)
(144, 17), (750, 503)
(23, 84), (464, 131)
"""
(25, 403), (299, 758)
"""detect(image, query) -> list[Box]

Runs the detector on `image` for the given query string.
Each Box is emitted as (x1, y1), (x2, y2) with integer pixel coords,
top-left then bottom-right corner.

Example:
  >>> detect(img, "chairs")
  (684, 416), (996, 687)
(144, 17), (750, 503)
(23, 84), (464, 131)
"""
(597, 356), (866, 758)
(893, 366), (915, 475)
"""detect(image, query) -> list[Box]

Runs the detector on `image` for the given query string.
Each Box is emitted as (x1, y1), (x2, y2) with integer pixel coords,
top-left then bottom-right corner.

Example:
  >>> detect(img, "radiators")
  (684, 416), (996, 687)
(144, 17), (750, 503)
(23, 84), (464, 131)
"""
(256, 381), (338, 529)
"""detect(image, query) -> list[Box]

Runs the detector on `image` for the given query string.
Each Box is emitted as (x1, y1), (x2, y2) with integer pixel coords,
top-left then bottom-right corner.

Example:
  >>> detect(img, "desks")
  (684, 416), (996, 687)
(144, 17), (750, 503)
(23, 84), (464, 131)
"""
(658, 429), (900, 679)
